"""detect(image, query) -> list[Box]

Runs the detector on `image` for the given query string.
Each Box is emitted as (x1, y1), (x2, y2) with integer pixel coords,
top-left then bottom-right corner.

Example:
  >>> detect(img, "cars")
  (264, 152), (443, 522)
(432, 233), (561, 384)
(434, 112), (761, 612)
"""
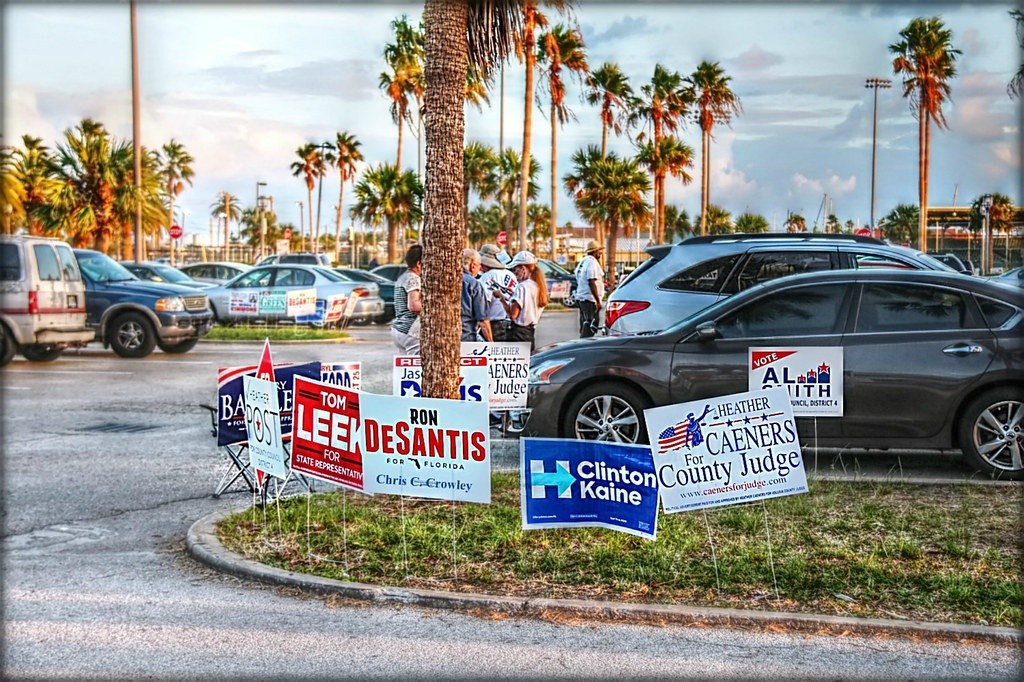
(257, 254), (331, 265)
(126, 261), (219, 292)
(181, 261), (252, 287)
(334, 266), (397, 326)
(200, 265), (386, 329)
(501, 270), (1024, 479)
(535, 257), (580, 308)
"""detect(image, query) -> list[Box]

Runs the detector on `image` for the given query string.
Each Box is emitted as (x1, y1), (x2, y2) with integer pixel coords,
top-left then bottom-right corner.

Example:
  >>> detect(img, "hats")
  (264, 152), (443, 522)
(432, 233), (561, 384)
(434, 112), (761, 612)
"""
(477, 244), (505, 269)
(505, 250), (538, 269)
(585, 241), (605, 253)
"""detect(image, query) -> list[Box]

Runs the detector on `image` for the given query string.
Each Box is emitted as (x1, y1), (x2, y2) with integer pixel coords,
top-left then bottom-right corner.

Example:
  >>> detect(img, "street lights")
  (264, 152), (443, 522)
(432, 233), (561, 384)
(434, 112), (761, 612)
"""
(866, 76), (893, 239)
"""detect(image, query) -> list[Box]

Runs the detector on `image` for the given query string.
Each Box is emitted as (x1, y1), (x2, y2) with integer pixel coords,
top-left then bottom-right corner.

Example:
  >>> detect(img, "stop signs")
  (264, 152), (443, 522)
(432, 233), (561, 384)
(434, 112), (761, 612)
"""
(169, 225), (182, 239)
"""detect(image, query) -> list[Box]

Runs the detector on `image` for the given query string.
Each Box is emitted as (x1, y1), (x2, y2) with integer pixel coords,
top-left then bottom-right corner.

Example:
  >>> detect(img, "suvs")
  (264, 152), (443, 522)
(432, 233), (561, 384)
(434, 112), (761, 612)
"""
(1, 231), (95, 370)
(603, 234), (961, 340)
(71, 247), (212, 356)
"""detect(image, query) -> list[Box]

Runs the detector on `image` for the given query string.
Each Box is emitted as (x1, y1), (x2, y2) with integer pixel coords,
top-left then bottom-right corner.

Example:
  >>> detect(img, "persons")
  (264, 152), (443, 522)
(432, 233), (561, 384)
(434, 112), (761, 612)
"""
(387, 243), (422, 358)
(458, 247), (495, 343)
(574, 240), (607, 337)
(477, 244), (520, 341)
(492, 250), (550, 352)
